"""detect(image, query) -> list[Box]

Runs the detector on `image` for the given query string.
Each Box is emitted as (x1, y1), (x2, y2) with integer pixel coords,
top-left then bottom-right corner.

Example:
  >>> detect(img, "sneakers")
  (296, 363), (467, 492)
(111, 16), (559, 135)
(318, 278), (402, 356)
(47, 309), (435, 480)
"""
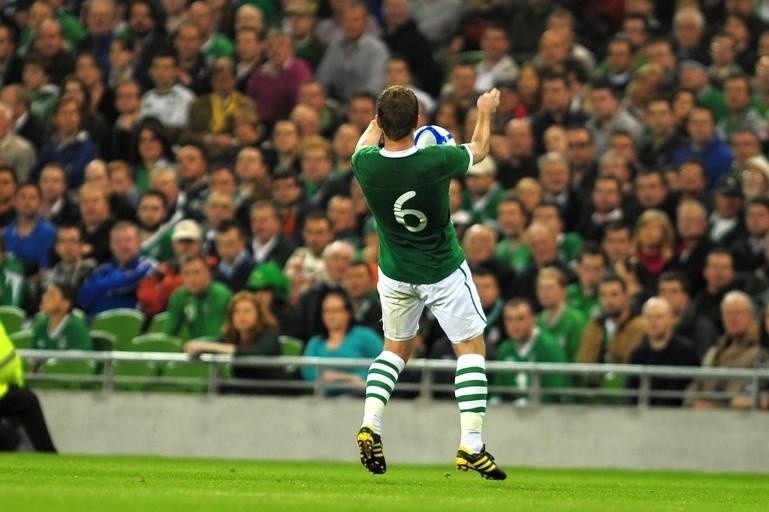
(455, 444), (506, 480)
(356, 425), (387, 475)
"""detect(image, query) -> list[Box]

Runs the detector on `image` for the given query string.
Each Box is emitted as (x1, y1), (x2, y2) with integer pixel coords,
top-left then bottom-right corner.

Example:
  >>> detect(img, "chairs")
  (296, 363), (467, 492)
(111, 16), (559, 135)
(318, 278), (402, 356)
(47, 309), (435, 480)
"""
(0, 301), (323, 398)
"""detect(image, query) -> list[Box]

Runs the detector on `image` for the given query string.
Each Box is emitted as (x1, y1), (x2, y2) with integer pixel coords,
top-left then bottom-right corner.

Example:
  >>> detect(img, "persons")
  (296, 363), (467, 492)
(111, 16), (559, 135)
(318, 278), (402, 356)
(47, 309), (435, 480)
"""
(0, 324), (58, 455)
(353, 80), (507, 480)
(1, 0), (768, 410)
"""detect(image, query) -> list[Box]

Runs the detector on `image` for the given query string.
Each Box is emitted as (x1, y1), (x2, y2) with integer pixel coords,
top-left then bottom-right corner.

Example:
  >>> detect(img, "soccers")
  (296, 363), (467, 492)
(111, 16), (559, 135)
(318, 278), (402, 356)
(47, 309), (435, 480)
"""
(413, 126), (456, 149)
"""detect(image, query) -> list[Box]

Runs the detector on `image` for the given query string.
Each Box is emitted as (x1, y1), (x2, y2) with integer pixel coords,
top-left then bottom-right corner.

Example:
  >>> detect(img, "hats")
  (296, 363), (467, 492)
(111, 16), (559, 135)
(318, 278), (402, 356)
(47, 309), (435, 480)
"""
(711, 173), (743, 197)
(170, 218), (202, 243)
